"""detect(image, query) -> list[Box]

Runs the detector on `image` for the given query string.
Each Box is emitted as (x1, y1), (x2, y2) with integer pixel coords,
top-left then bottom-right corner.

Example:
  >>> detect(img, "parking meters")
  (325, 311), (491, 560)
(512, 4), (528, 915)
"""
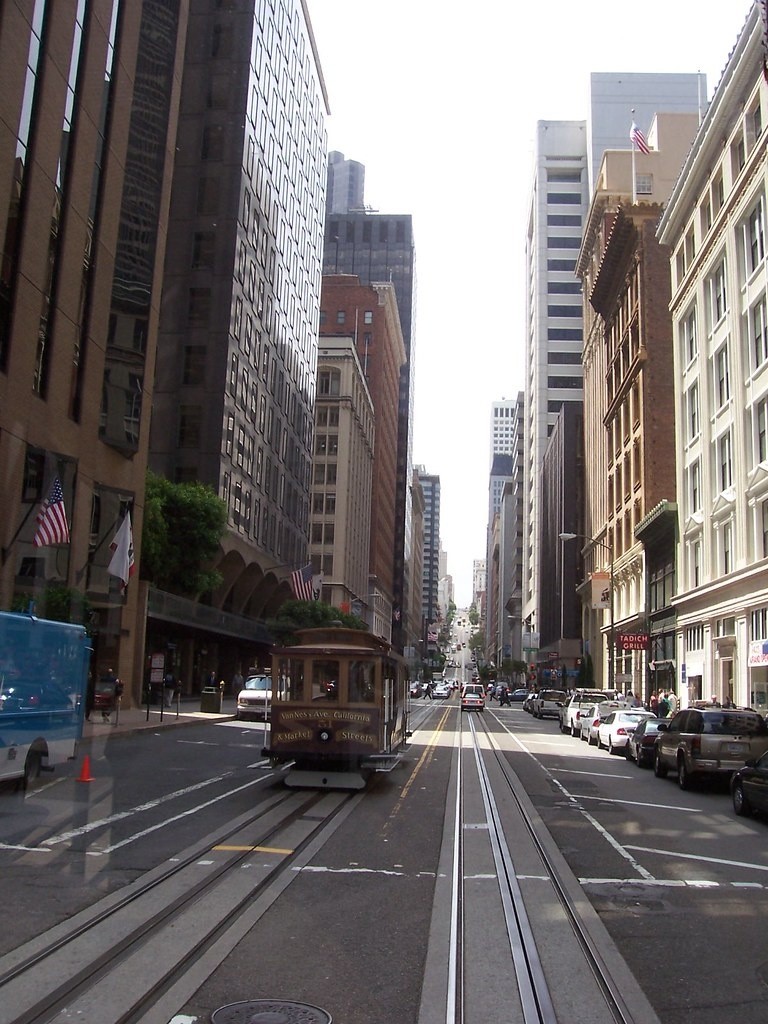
(114, 682), (124, 726)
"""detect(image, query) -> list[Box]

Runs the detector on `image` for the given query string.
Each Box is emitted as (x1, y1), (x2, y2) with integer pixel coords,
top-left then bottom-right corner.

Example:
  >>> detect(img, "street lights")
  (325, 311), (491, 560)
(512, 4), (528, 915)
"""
(558, 532), (614, 701)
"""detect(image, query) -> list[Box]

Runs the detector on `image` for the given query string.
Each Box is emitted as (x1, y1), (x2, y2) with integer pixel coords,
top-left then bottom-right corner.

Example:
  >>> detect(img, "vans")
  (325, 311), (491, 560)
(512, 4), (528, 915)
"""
(0, 602), (93, 787)
(409, 680), (423, 698)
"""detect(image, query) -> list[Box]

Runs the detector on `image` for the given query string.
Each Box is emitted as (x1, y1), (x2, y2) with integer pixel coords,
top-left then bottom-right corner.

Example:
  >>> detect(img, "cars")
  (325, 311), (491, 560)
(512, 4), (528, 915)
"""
(489, 679), (634, 746)
(625, 717), (676, 767)
(421, 682), (429, 692)
(729, 750), (768, 819)
(597, 709), (658, 755)
(430, 607), (486, 714)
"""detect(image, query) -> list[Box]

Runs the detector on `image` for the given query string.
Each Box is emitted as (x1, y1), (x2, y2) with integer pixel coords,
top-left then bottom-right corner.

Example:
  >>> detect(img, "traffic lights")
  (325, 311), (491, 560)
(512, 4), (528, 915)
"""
(531, 674), (536, 680)
(530, 663), (534, 669)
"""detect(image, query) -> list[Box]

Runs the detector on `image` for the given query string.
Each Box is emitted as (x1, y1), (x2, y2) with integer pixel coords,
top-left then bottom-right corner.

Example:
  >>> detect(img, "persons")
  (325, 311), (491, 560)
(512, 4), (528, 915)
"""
(423, 685), (433, 699)
(722, 695), (737, 710)
(616, 688), (643, 707)
(84, 674), (95, 721)
(411, 688), (422, 698)
(432, 682), (435, 688)
(165, 670), (175, 708)
(500, 686), (511, 706)
(456, 680), (458, 689)
(650, 688), (677, 718)
(205, 670), (217, 691)
(99, 668), (125, 724)
(460, 683), (464, 698)
(489, 686), (498, 701)
(444, 680), (448, 685)
(711, 695), (721, 708)
(232, 671), (244, 703)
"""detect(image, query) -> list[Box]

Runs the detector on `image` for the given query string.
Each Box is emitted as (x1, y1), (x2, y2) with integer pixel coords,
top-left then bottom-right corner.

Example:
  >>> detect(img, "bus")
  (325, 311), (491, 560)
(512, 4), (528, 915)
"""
(261, 626), (414, 790)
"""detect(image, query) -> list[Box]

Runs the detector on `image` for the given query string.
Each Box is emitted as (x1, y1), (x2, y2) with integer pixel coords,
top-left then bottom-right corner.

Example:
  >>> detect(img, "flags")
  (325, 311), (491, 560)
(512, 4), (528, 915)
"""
(292, 562), (315, 601)
(339, 601), (351, 614)
(34, 473), (70, 547)
(311, 573), (323, 603)
(107, 510), (135, 591)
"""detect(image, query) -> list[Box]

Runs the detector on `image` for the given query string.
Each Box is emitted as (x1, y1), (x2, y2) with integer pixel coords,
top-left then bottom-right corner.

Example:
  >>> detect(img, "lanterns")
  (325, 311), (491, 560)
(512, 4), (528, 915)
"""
(530, 684), (536, 689)
(530, 663), (536, 670)
(530, 673), (536, 680)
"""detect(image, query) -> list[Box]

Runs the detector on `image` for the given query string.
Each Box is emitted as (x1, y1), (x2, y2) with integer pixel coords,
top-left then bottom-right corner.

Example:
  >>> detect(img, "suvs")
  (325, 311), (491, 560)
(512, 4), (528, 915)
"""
(653, 696), (768, 791)
(236, 674), (289, 721)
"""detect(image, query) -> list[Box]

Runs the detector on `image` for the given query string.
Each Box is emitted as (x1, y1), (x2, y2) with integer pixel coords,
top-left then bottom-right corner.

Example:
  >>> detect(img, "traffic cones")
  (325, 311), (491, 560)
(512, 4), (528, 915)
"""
(74, 754), (95, 782)
